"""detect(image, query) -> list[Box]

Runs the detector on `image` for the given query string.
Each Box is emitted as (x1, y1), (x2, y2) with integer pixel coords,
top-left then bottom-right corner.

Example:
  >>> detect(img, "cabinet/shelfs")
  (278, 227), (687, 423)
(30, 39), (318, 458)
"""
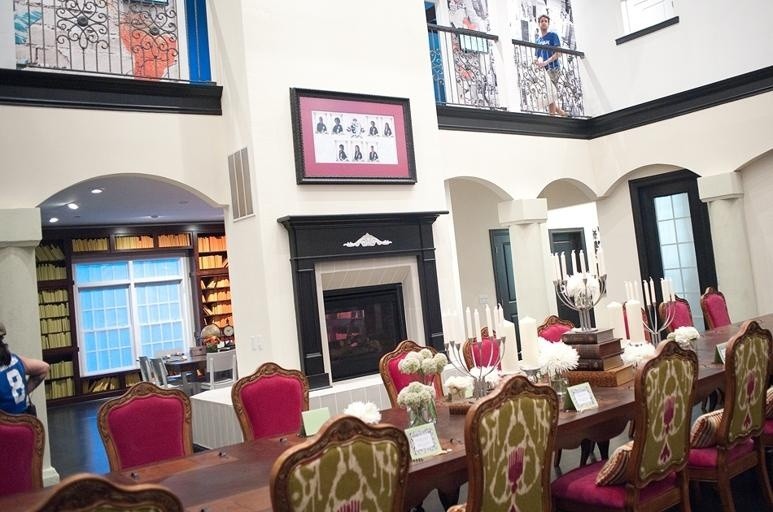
(189, 223), (233, 344)
(35, 235), (81, 406)
(68, 221), (193, 262)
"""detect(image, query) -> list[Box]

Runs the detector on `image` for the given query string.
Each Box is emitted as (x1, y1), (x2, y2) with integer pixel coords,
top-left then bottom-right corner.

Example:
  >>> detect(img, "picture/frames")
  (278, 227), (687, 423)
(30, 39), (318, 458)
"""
(290, 87), (417, 185)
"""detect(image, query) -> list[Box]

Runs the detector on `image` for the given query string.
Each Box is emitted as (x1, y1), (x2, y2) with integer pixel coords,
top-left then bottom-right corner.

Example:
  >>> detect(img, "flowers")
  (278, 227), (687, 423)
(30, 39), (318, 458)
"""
(562, 273), (600, 297)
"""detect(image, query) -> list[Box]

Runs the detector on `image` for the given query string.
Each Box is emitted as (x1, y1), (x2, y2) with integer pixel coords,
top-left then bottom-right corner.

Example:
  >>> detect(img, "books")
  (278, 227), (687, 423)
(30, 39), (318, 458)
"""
(562, 329), (637, 388)
(34, 231), (233, 401)
(449, 400), (475, 416)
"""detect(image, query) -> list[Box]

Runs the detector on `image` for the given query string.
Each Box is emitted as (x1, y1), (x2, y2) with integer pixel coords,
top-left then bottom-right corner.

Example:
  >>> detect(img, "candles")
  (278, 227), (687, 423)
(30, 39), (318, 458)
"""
(608, 277), (676, 308)
(549, 250), (607, 284)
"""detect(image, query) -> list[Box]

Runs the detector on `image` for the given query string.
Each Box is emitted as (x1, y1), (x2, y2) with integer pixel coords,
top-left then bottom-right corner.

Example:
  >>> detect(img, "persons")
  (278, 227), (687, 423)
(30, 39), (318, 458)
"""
(354, 145), (362, 161)
(317, 117), (327, 132)
(333, 118), (343, 133)
(384, 122), (392, 136)
(369, 146), (378, 161)
(369, 121), (378, 137)
(339, 144), (348, 161)
(1, 323), (50, 417)
(534, 15), (569, 118)
